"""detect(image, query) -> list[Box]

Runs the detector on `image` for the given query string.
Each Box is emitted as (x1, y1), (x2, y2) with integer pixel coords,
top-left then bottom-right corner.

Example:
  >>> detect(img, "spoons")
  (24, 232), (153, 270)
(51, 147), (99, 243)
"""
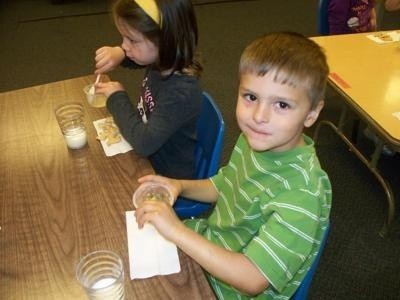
(89, 72), (101, 103)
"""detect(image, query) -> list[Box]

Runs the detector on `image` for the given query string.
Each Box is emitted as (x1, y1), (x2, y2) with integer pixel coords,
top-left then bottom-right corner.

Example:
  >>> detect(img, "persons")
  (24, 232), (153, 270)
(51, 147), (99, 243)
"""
(328, 1), (376, 35)
(94, 0), (202, 181)
(132, 31), (331, 300)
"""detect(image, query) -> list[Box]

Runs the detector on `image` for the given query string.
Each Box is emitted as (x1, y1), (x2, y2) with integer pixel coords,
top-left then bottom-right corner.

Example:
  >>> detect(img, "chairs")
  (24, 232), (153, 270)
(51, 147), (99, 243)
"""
(173, 91), (332, 299)
(317, 1), (330, 35)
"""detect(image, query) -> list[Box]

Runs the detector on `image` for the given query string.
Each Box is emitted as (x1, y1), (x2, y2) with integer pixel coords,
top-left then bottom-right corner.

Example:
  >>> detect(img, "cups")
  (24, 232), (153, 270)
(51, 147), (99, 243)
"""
(83, 83), (108, 109)
(54, 101), (88, 150)
(76, 250), (125, 300)
(132, 181), (174, 214)
